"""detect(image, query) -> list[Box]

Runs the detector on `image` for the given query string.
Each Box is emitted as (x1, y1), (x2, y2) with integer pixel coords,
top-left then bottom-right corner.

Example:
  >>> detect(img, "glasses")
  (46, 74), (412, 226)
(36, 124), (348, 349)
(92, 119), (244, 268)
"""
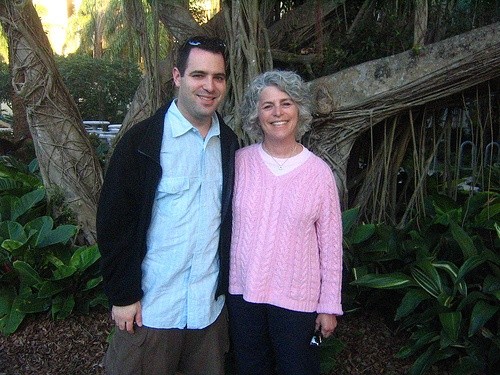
(310, 328), (323, 347)
(179, 37), (228, 56)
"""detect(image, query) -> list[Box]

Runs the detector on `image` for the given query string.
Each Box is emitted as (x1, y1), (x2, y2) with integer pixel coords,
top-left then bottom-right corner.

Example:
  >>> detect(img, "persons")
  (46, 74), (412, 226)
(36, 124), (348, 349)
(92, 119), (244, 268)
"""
(95, 35), (242, 375)
(229, 71), (343, 375)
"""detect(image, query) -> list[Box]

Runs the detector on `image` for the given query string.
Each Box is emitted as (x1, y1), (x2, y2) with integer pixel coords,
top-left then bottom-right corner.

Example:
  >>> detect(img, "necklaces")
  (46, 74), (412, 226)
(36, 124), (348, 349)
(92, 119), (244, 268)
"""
(263, 137), (299, 172)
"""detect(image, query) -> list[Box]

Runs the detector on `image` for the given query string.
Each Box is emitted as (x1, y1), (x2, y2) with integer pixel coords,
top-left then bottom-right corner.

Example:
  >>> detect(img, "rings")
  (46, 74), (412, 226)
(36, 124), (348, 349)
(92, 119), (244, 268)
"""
(330, 330), (334, 334)
